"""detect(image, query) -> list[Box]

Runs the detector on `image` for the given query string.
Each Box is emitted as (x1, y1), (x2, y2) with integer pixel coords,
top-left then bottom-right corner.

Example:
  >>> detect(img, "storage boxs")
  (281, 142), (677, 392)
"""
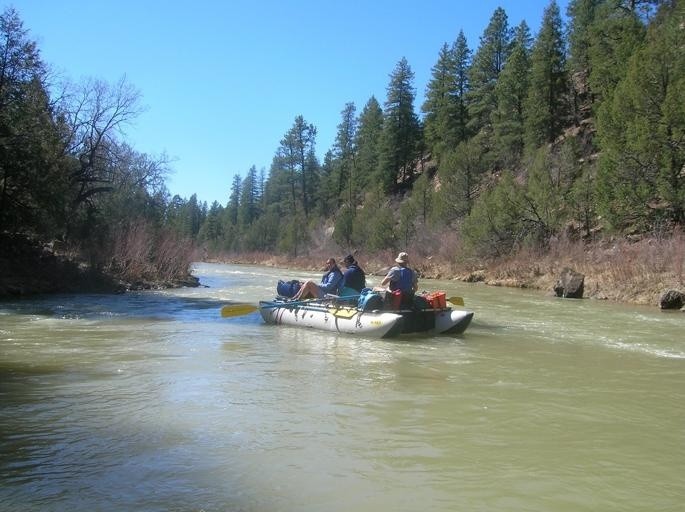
(426, 292), (446, 309)
(378, 291), (401, 311)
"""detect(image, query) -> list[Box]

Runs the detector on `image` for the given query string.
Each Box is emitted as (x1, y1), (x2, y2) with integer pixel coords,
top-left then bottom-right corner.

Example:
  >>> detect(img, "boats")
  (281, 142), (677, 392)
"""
(257, 291), (475, 342)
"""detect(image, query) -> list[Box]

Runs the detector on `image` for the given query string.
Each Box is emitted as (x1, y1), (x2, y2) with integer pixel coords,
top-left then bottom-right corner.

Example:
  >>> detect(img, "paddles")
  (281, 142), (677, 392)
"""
(221, 294), (362, 318)
(260, 301), (357, 320)
(446, 297), (464, 306)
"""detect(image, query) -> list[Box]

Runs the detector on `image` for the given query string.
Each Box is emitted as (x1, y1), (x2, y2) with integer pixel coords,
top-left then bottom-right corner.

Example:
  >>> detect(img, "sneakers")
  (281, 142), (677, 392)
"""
(287, 297), (303, 303)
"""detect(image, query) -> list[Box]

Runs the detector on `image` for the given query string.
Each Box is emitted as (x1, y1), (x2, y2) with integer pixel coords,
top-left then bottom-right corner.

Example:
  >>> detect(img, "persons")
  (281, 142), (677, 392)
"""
(381, 252), (418, 296)
(291, 254), (365, 300)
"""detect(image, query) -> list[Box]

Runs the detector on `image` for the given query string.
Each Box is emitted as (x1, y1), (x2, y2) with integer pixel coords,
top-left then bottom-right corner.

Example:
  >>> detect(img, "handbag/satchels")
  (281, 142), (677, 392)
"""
(358, 288), (383, 310)
(277, 279), (299, 297)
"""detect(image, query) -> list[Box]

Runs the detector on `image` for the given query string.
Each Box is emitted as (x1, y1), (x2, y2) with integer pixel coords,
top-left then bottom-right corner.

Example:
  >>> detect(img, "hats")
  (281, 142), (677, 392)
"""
(395, 252), (409, 264)
(338, 255), (358, 264)
